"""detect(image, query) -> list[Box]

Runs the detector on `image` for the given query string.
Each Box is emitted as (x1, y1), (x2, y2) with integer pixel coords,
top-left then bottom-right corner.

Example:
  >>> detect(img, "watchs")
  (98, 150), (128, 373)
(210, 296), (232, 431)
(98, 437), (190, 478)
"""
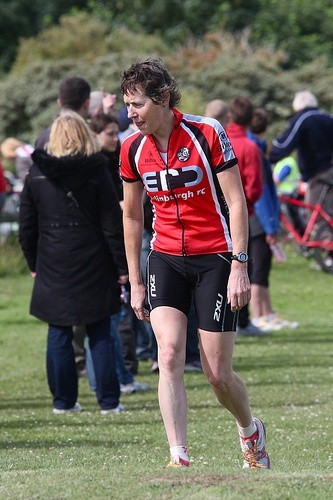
(230, 252), (249, 264)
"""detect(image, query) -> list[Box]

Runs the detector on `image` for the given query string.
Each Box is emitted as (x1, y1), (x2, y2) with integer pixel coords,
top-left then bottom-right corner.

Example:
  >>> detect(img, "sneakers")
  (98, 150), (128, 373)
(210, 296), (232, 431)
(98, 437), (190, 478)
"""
(165, 456), (190, 471)
(99, 404), (124, 414)
(237, 323), (264, 339)
(53, 402), (83, 414)
(183, 358), (203, 373)
(239, 417), (270, 472)
(255, 312), (301, 331)
(119, 380), (151, 394)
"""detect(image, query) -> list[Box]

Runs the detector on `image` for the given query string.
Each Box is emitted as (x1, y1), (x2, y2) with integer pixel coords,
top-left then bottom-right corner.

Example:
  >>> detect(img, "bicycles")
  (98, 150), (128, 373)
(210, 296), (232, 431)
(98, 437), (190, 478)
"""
(272, 175), (333, 274)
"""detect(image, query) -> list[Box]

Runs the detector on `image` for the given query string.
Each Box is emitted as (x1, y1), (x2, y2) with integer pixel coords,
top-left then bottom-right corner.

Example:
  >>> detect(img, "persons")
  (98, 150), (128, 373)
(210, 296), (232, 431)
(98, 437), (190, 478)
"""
(264, 91), (333, 270)
(33, 77), (241, 395)
(247, 107), (299, 332)
(18, 110), (129, 415)
(225, 96), (263, 337)
(119, 57), (270, 470)
(273, 156), (302, 195)
(1, 137), (35, 179)
